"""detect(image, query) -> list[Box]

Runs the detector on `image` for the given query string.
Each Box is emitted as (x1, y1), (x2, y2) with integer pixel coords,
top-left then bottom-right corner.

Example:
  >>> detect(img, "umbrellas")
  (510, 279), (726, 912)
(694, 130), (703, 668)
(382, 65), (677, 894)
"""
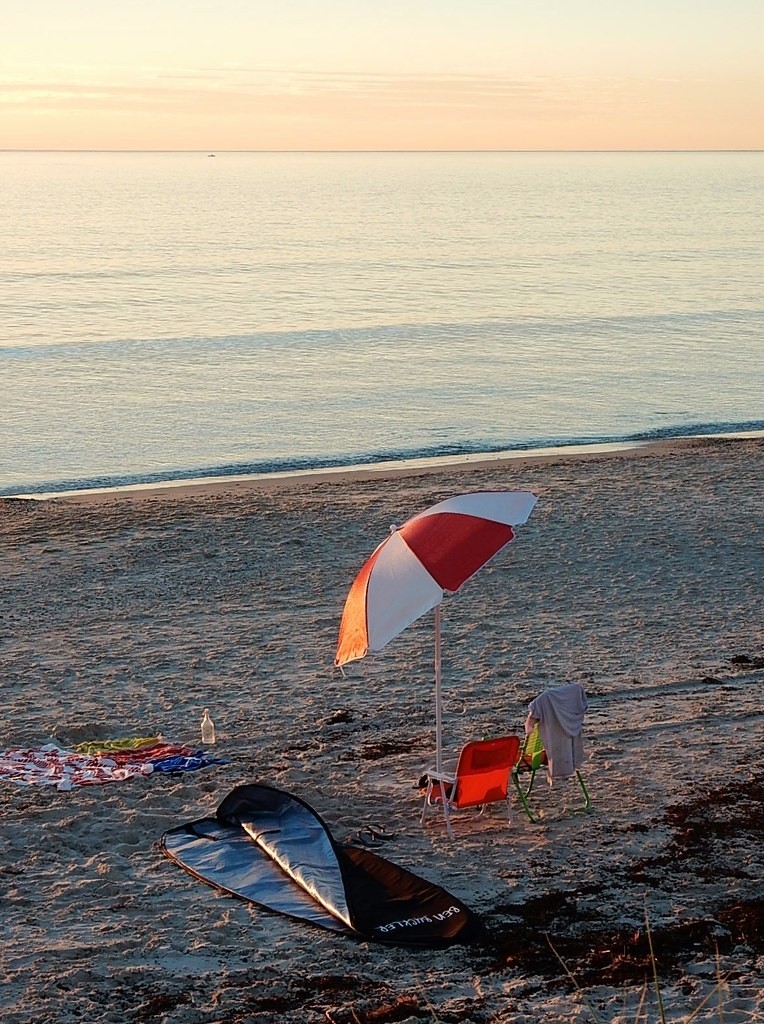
(335, 489), (538, 775)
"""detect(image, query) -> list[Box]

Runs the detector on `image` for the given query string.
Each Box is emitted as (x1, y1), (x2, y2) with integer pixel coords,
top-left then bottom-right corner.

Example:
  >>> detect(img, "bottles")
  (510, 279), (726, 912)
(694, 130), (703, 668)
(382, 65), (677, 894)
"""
(201, 708), (215, 743)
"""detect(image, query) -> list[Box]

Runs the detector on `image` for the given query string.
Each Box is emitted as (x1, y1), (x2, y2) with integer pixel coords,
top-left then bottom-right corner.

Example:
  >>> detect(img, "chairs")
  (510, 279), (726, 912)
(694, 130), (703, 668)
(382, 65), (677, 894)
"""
(415, 735), (522, 837)
(511, 685), (590, 824)
